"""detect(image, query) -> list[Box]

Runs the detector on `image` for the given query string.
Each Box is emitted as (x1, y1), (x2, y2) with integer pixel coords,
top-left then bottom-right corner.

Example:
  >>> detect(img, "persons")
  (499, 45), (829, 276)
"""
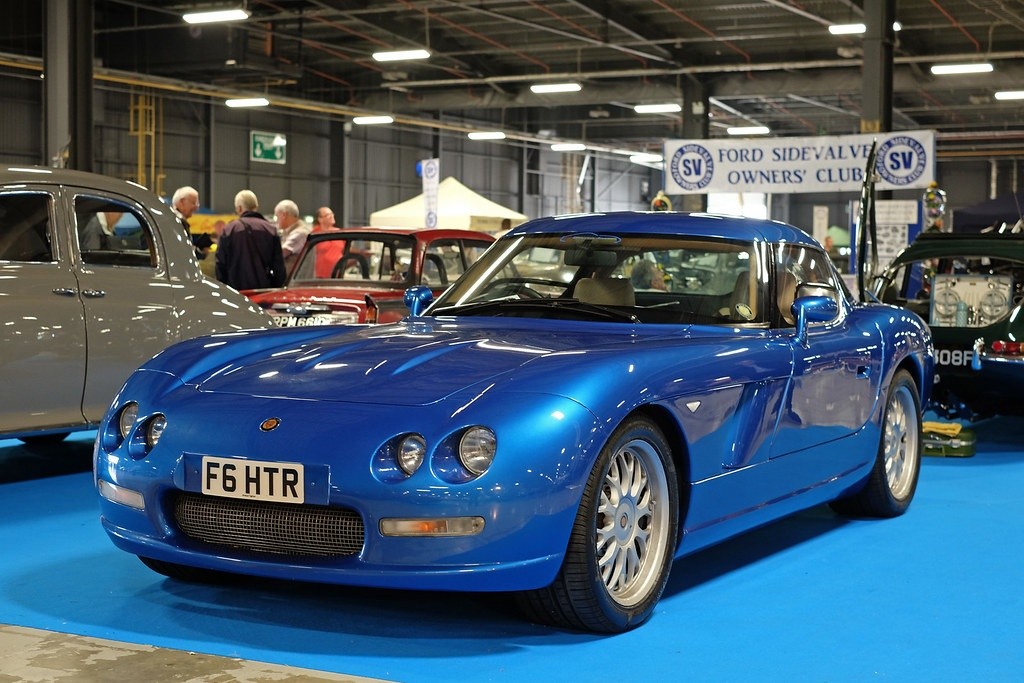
(171, 186), (345, 291)
(823, 237), (842, 273)
(78, 212), (124, 250)
(631, 259), (666, 293)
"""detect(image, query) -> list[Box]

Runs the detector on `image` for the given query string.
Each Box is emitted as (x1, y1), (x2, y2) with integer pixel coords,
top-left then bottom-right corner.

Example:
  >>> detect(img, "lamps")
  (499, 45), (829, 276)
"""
(531, 50), (582, 94)
(182, 0), (251, 25)
(728, 126), (770, 135)
(829, 6), (901, 34)
(225, 80), (269, 108)
(931, 22), (996, 75)
(373, 7), (432, 62)
(995, 91), (1024, 100)
(551, 123), (586, 151)
(468, 108), (506, 140)
(634, 74), (682, 114)
(353, 88), (392, 124)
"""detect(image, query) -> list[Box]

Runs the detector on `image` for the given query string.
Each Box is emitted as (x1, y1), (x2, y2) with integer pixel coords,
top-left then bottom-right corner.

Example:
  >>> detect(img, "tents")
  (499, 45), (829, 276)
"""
(814, 226), (850, 246)
(370, 176), (528, 277)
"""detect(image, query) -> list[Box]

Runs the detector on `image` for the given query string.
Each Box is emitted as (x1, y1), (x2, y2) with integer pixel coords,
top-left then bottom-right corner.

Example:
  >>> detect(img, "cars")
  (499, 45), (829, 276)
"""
(93, 211), (934, 634)
(0, 167), (281, 438)
(240, 228), (526, 328)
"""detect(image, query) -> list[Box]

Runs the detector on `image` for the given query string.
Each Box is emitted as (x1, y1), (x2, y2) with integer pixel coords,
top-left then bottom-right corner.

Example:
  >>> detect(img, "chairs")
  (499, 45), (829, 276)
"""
(574, 277), (635, 306)
(728, 270), (797, 322)
